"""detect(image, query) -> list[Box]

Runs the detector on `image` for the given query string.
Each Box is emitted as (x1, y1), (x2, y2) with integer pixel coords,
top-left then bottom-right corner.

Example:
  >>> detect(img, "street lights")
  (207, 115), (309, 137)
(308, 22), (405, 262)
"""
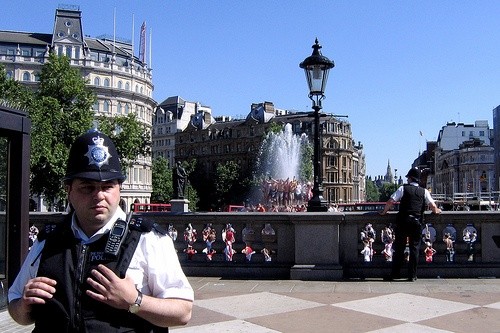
(299, 36), (335, 212)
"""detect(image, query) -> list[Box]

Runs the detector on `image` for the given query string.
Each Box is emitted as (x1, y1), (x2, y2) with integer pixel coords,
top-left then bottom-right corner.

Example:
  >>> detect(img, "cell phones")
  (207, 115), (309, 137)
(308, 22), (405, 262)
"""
(105, 211), (133, 256)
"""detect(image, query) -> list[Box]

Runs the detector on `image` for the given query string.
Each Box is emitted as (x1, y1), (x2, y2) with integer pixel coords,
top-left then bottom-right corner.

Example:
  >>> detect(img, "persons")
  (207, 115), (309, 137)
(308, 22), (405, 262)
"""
(8, 121), (194, 333)
(167, 223), (270, 261)
(173, 160), (189, 199)
(360, 223), (477, 263)
(28, 225), (39, 253)
(240, 175), (343, 213)
(377, 166), (442, 282)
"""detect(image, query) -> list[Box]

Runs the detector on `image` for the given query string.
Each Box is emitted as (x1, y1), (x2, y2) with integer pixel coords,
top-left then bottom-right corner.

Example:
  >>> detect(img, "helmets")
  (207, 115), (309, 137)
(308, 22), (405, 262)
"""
(63, 128), (124, 184)
(405, 167), (421, 181)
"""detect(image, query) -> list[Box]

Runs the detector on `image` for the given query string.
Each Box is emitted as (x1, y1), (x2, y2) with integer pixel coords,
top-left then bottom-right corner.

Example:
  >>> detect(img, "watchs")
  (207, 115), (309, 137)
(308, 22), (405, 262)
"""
(128, 289), (143, 314)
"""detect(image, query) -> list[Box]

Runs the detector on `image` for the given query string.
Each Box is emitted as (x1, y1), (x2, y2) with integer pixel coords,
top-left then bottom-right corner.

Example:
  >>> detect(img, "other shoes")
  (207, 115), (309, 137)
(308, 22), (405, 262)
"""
(408, 275), (417, 282)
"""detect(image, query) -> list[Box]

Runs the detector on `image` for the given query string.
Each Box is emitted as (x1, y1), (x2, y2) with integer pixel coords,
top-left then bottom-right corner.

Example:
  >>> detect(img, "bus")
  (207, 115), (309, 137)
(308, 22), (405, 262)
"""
(130, 202), (171, 212)
